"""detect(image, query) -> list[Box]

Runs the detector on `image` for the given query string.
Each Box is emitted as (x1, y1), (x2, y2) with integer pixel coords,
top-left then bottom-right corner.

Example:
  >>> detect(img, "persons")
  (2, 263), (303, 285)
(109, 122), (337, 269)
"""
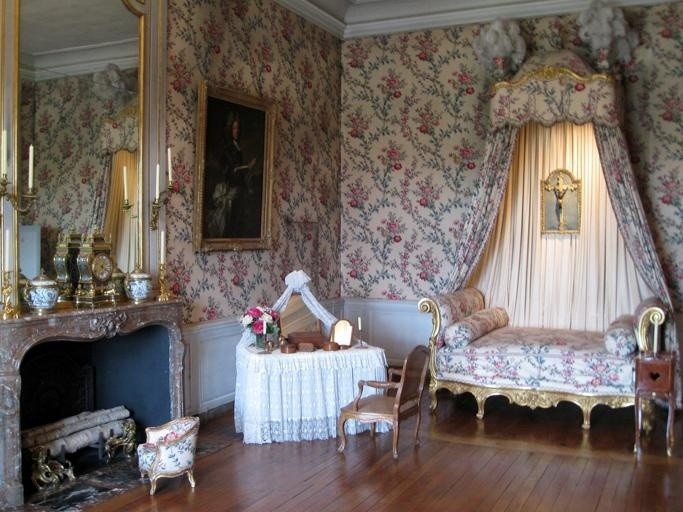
(205, 111), (261, 237)
(545, 176), (578, 230)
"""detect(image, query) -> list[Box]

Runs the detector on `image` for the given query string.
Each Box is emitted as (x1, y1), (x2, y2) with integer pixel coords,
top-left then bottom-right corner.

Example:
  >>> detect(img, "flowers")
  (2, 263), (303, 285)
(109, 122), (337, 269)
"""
(239, 306), (280, 334)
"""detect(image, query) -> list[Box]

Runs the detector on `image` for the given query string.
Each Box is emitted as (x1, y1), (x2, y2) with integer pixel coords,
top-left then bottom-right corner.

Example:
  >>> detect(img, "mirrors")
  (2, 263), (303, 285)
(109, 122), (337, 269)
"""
(329, 319), (354, 347)
(0, 0), (151, 312)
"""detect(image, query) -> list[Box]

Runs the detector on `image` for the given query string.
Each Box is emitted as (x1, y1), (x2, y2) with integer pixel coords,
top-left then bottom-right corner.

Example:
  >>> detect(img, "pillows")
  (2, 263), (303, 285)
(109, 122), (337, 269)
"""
(444, 306), (509, 347)
(604, 314), (637, 356)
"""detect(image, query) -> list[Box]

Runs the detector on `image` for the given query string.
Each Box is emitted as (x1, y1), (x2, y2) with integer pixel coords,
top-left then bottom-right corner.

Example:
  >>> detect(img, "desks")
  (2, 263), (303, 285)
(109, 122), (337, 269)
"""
(632, 351), (675, 458)
(234, 345), (394, 445)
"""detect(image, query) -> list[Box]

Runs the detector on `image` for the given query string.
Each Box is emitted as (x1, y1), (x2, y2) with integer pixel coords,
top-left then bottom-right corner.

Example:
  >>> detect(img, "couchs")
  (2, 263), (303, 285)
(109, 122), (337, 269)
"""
(417, 286), (667, 428)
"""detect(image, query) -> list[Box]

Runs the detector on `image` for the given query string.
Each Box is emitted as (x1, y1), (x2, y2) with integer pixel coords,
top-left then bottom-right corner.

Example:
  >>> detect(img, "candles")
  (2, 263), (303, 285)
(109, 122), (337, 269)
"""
(28, 144), (34, 189)
(653, 317), (659, 353)
(1, 130), (6, 173)
(156, 163), (159, 200)
(168, 148), (173, 181)
(123, 165), (128, 200)
(358, 316), (362, 330)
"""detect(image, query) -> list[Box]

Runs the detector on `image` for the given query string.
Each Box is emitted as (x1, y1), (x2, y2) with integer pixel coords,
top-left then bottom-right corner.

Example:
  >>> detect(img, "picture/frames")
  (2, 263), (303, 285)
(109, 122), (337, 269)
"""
(539, 167), (582, 234)
(256, 333), (265, 348)
(193, 82), (279, 252)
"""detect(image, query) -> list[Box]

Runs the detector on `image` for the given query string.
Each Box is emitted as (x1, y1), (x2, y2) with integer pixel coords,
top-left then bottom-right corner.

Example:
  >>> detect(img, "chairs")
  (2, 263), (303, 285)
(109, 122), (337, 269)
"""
(336, 345), (431, 459)
(136, 416), (200, 496)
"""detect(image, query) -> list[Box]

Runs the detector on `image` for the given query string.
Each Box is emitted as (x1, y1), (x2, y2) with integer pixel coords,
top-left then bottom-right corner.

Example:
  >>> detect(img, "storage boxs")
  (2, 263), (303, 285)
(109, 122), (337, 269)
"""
(289, 332), (323, 349)
